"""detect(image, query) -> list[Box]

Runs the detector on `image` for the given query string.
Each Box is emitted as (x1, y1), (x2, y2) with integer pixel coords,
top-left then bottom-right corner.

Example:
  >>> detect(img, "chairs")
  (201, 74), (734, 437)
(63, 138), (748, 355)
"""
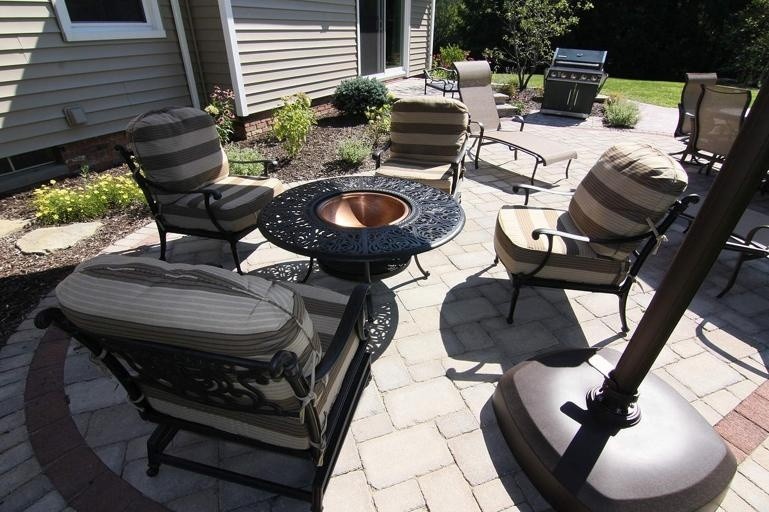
(370, 95), (472, 203)
(678, 83), (752, 177)
(452, 59), (578, 185)
(113, 106), (286, 277)
(493, 141), (701, 333)
(423, 67), (459, 99)
(668, 72), (718, 169)
(33, 252), (375, 512)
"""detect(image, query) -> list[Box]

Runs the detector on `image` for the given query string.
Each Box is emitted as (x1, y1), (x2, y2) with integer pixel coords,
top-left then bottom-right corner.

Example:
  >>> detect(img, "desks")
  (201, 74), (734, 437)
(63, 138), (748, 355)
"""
(257, 173), (467, 286)
